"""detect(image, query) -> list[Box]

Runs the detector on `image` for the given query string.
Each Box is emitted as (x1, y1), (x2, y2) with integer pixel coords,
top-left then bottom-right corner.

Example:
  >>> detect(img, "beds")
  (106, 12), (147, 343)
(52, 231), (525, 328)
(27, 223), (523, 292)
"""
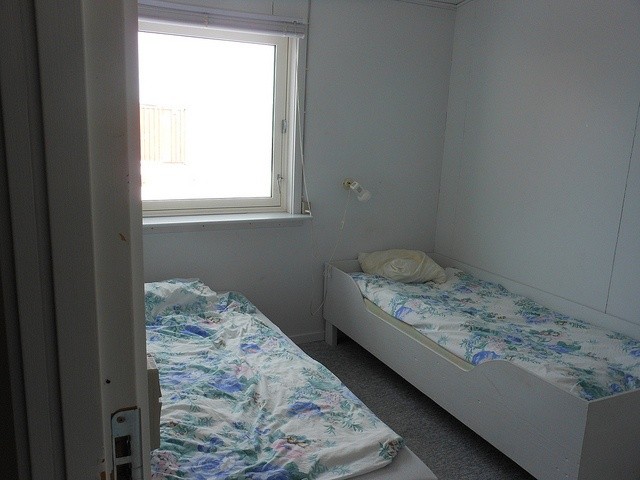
(324, 250), (640, 480)
(142, 279), (438, 480)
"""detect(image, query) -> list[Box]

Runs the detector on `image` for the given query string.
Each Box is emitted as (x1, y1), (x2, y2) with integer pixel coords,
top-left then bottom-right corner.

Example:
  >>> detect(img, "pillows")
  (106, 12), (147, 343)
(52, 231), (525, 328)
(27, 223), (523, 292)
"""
(356, 249), (446, 283)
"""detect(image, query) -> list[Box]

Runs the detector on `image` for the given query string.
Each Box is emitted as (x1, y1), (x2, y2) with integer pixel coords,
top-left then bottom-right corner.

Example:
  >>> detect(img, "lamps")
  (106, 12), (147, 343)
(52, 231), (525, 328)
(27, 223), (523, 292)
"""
(343, 177), (370, 203)
